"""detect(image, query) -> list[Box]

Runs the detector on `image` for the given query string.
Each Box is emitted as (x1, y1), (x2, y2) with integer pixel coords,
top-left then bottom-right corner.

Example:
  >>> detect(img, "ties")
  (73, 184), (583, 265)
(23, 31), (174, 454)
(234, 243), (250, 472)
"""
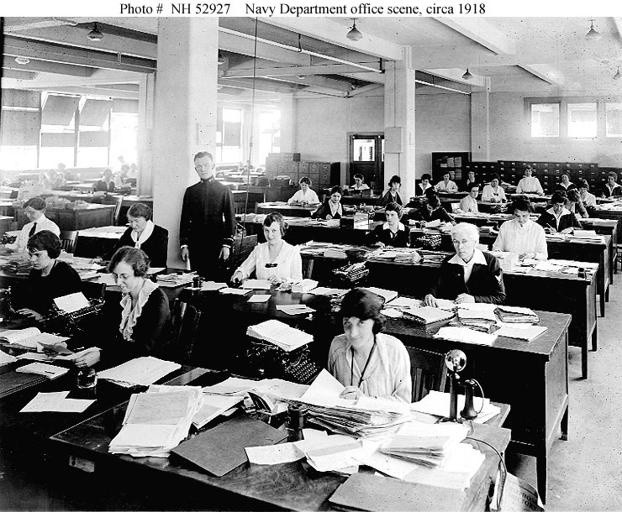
(29, 223), (36, 236)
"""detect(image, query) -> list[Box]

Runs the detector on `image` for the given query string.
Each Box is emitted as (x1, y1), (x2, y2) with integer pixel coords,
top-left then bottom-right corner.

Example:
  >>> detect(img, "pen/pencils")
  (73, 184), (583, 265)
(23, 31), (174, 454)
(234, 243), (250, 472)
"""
(546, 222), (553, 229)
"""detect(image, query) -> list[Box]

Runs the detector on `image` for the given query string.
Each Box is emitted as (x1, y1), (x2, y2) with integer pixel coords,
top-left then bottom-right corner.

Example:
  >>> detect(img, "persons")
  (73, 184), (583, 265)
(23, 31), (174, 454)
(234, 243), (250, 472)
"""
(0, 152), (622, 407)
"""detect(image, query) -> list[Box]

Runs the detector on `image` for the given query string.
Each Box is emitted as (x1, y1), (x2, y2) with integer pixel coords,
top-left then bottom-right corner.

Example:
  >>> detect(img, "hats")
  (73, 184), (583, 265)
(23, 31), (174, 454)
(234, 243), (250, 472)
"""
(341, 289), (383, 320)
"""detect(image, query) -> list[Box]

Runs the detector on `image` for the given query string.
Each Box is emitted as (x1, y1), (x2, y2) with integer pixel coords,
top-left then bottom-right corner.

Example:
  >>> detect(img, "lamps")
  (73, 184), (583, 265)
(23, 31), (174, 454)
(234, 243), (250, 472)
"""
(87, 22), (102, 41)
(586, 20), (600, 38)
(462, 68), (472, 79)
(347, 19), (362, 40)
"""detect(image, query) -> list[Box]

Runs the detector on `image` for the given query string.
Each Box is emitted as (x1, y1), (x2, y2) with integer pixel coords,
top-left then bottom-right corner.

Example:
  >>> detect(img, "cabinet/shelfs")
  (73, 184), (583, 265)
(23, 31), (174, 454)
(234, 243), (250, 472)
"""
(431, 151), (622, 194)
(266, 152), (340, 186)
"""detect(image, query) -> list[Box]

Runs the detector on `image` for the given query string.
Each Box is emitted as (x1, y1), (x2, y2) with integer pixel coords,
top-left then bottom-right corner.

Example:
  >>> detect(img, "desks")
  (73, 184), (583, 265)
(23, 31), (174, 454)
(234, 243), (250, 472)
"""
(0, 182), (622, 512)
